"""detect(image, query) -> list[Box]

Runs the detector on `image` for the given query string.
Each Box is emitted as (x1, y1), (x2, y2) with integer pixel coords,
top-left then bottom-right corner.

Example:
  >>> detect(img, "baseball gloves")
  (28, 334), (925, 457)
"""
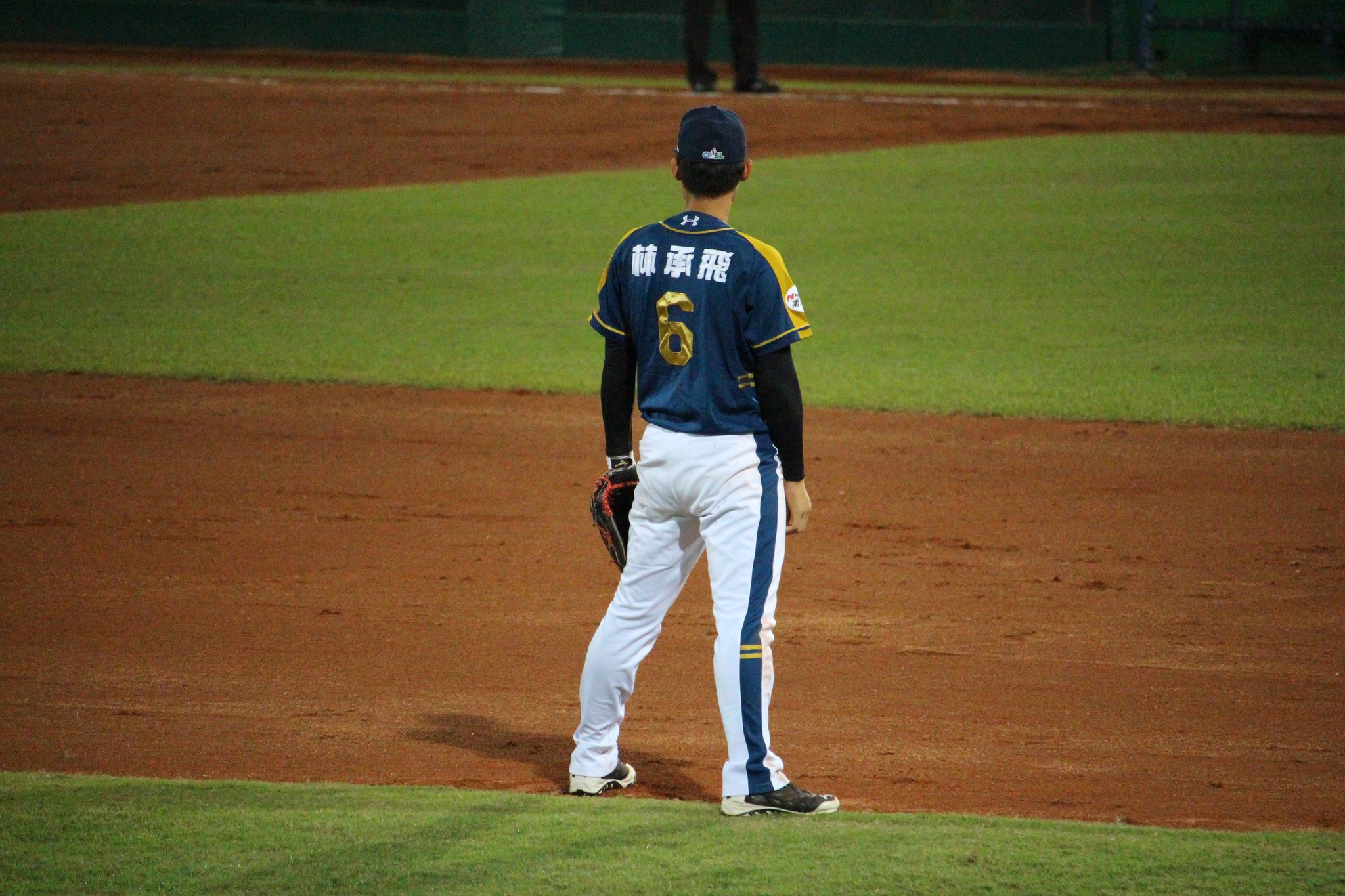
(590, 467), (639, 572)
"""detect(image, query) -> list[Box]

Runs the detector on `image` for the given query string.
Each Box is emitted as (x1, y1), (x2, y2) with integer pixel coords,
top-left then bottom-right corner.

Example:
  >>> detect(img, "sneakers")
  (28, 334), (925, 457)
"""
(569, 760), (634, 795)
(721, 782), (840, 815)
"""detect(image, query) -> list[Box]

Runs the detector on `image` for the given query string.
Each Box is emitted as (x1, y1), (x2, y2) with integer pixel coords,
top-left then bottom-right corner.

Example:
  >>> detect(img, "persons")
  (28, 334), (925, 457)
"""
(679, 0), (781, 94)
(566, 98), (841, 814)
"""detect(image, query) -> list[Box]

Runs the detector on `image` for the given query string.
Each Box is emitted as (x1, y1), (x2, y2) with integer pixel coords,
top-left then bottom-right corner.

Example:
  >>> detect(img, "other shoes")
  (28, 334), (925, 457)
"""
(690, 81), (715, 93)
(733, 78), (780, 92)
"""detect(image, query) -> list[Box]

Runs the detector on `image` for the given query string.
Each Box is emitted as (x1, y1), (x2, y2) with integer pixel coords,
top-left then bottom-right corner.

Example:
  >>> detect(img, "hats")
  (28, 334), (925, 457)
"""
(677, 105), (746, 163)
